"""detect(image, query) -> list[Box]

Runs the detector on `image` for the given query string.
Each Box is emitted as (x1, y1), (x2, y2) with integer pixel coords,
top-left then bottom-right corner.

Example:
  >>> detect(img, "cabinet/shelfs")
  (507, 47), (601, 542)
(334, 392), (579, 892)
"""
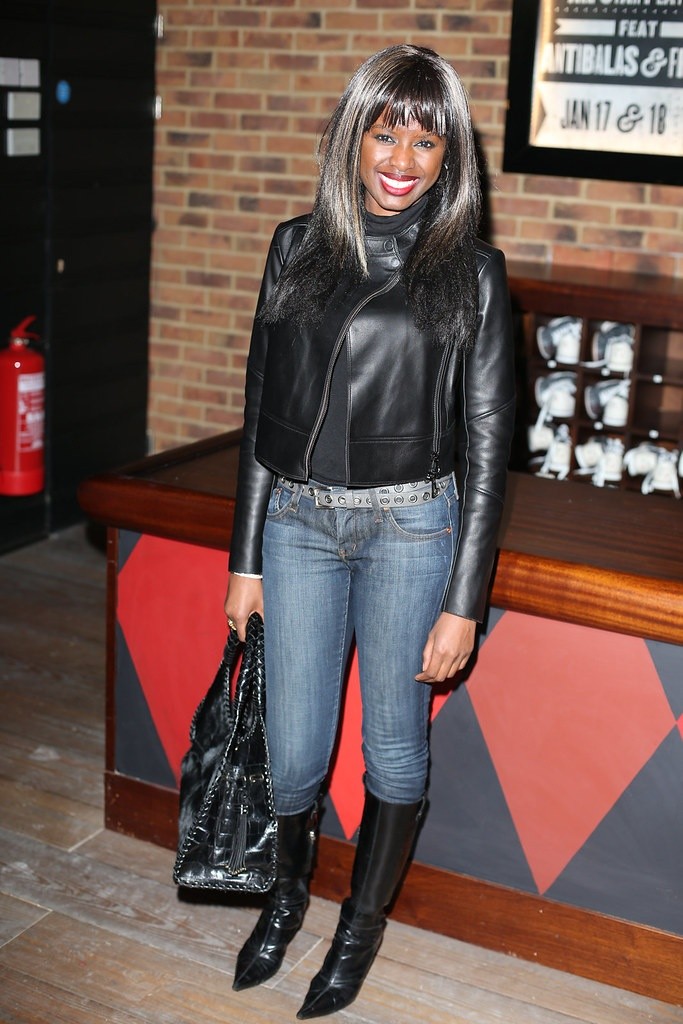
(507, 259), (683, 501)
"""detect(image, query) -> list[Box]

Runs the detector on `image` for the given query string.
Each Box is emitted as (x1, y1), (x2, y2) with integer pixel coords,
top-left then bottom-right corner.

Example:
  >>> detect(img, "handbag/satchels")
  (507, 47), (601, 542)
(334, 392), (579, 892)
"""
(173, 615), (276, 894)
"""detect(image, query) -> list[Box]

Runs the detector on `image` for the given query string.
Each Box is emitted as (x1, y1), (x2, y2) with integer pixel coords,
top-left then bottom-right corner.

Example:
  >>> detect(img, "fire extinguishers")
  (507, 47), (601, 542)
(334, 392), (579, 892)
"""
(0, 316), (50, 496)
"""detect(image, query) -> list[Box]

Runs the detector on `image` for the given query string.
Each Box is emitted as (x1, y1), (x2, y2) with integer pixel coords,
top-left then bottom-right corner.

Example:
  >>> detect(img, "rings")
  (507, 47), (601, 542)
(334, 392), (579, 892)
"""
(228, 618), (235, 630)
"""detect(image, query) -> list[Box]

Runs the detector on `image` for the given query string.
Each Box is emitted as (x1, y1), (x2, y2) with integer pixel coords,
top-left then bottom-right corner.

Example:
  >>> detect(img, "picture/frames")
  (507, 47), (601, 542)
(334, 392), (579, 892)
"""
(502, 0), (683, 187)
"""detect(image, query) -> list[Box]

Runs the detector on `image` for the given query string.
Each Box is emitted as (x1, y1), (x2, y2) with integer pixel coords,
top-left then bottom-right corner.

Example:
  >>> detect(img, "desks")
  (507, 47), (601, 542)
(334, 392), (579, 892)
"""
(81, 430), (683, 1008)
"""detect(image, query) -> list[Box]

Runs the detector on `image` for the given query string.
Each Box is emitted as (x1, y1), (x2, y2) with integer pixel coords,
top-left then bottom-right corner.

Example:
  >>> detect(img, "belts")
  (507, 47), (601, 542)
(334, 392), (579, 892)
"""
(279, 477), (451, 508)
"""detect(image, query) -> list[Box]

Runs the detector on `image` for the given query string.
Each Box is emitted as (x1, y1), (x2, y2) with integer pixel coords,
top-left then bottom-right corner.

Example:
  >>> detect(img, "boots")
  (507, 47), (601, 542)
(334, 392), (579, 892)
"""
(298, 770), (428, 1018)
(232, 804), (320, 992)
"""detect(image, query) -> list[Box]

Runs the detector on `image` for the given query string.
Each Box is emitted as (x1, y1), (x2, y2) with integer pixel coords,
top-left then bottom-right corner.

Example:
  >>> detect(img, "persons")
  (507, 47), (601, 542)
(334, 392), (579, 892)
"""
(224, 43), (518, 1019)
